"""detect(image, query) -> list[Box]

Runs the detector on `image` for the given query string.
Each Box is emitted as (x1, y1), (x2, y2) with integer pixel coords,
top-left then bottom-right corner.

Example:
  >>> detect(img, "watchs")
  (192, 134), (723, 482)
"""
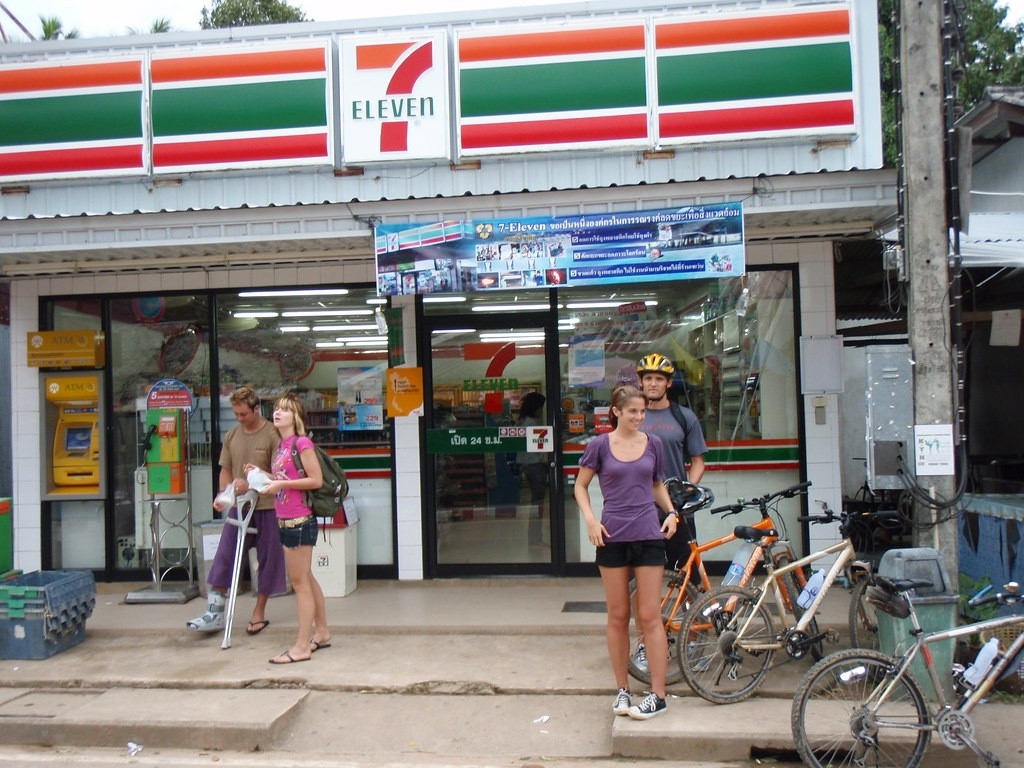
(666, 510), (679, 517)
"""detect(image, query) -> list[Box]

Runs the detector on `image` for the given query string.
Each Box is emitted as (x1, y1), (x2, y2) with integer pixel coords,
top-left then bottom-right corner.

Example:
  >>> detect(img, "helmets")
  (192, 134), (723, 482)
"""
(637, 354), (674, 377)
(664, 475), (715, 516)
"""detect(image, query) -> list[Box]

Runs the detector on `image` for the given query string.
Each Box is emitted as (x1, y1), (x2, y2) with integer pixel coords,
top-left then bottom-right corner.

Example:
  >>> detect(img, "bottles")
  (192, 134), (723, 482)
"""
(720, 544), (754, 586)
(797, 568), (826, 608)
(964, 637), (999, 684)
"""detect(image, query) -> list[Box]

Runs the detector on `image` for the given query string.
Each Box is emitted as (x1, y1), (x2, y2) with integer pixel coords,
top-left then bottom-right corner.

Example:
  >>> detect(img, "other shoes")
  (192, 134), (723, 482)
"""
(531, 541), (550, 550)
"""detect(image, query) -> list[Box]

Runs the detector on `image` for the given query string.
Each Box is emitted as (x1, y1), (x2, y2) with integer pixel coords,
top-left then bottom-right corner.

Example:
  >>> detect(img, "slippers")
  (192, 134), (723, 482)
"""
(247, 618), (269, 634)
(310, 638), (330, 651)
(269, 650), (311, 664)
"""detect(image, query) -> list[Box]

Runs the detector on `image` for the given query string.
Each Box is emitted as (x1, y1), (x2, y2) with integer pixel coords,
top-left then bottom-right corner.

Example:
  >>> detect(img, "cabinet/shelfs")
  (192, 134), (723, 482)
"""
(304, 407), (391, 447)
(675, 271), (799, 443)
(445, 412), (491, 512)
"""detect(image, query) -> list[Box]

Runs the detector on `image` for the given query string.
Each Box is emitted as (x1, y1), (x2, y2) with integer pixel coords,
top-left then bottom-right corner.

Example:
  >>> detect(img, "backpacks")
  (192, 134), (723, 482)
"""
(291, 435), (349, 518)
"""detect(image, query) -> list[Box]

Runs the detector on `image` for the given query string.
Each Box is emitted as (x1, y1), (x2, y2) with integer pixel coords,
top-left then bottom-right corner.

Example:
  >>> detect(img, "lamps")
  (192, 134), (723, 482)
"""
(231, 289), (660, 349)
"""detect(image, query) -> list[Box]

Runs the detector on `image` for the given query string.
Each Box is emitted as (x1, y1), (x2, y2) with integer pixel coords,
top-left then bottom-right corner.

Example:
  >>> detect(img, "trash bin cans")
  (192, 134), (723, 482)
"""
(310, 496), (357, 597)
(193, 519), (243, 599)
(249, 546), (294, 597)
(874, 548), (959, 702)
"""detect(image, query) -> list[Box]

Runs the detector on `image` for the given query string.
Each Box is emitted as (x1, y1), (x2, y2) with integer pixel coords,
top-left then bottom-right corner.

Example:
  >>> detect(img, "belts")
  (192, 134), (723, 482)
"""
(278, 515), (312, 529)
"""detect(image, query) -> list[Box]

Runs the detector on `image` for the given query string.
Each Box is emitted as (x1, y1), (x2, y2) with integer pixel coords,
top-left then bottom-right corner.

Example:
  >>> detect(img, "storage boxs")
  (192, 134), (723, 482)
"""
(0, 569), (98, 660)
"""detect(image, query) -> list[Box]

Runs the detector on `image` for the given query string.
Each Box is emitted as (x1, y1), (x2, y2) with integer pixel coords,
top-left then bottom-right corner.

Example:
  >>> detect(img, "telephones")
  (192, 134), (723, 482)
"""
(144, 415), (176, 450)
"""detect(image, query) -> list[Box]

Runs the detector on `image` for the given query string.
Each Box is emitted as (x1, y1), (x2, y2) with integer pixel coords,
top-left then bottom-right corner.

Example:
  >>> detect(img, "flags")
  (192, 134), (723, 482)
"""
(671, 336), (707, 385)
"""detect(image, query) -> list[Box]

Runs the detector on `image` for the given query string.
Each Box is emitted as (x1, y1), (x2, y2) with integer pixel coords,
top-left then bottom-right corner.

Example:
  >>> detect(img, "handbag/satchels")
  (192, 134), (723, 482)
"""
(505, 444), (520, 476)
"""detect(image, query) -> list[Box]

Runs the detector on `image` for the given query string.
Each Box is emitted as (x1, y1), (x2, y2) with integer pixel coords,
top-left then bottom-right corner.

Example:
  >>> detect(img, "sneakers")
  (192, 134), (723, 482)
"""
(611, 686), (631, 715)
(628, 691), (668, 720)
(634, 644), (650, 672)
(689, 642), (709, 671)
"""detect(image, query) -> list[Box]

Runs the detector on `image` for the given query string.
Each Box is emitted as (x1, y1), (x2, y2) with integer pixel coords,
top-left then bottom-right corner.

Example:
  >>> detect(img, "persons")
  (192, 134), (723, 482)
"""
(434, 404), (458, 509)
(574, 386), (679, 720)
(514, 392), (551, 551)
(633, 354), (710, 674)
(243, 392), (332, 664)
(185, 386), (286, 634)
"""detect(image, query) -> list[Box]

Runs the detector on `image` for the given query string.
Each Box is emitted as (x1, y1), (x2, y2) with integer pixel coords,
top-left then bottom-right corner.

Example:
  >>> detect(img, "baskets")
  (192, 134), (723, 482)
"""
(978, 622), (1024, 694)
